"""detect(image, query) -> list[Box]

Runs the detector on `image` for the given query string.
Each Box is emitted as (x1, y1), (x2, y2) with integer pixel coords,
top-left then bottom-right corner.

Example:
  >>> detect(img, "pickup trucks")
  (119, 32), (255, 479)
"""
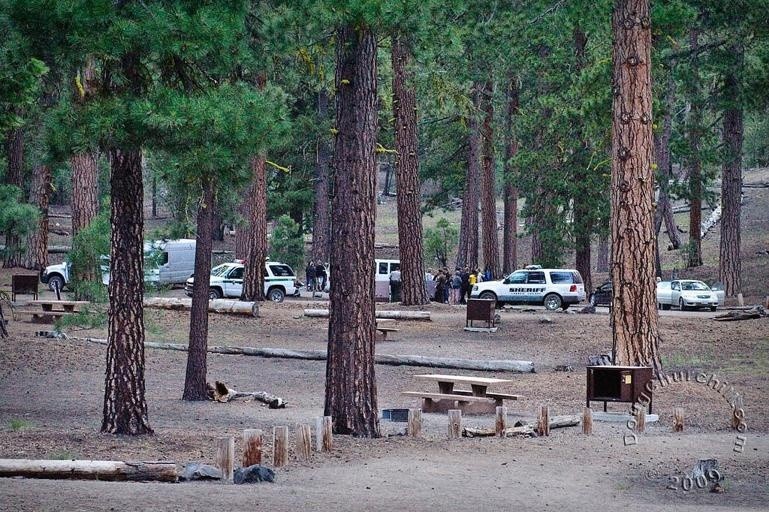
(656, 276), (726, 312)
(35, 248), (163, 291)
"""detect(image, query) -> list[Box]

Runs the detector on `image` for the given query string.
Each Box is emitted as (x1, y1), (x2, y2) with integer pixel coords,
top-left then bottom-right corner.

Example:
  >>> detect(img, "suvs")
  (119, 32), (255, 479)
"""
(182, 258), (304, 303)
(471, 263), (589, 314)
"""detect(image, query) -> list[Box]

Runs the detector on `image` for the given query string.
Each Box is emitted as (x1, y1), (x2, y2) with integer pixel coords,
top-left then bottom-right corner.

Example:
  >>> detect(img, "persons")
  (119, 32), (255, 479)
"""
(657, 276), (664, 309)
(389, 266), (486, 305)
(305, 259), (330, 292)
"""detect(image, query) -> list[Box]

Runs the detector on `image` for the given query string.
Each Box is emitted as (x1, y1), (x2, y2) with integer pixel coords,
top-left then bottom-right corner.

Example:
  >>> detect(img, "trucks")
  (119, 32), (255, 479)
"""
(139, 237), (197, 285)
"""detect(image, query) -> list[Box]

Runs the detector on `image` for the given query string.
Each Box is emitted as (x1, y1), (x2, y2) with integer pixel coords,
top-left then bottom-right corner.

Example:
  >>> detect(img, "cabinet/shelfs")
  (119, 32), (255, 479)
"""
(583, 364), (654, 416)
(466, 298), (496, 329)
(11, 273), (39, 301)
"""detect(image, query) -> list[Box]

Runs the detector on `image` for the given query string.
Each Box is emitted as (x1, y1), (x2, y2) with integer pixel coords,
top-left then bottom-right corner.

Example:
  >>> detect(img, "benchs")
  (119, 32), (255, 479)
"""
(52, 308), (97, 321)
(377, 328), (401, 341)
(19, 310), (73, 324)
(403, 390), (494, 414)
(452, 388), (527, 412)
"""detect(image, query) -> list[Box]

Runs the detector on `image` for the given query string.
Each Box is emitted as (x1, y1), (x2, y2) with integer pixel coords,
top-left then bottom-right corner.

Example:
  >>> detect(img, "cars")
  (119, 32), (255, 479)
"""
(588, 278), (612, 309)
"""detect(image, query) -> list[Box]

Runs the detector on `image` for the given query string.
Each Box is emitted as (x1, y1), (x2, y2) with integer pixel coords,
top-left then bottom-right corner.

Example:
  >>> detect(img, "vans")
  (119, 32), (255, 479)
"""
(373, 258), (401, 302)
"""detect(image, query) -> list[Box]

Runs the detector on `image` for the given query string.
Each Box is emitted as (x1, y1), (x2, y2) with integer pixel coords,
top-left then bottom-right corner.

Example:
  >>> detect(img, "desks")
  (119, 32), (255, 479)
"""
(27, 299), (90, 319)
(375, 318), (396, 336)
(413, 372), (512, 411)
